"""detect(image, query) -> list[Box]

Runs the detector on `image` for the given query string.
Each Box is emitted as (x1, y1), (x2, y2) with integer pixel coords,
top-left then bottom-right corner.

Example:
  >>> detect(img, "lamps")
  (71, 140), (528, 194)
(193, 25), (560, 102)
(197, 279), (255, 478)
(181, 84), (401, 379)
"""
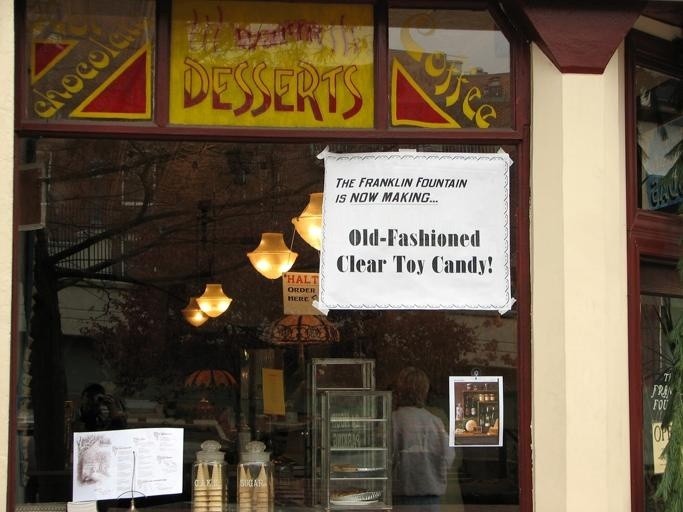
(178, 142), (323, 328)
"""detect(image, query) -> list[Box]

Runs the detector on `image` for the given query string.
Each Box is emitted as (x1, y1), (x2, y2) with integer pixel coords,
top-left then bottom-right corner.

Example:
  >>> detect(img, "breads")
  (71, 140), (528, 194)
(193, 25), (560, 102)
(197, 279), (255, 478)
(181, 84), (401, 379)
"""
(331, 487), (368, 498)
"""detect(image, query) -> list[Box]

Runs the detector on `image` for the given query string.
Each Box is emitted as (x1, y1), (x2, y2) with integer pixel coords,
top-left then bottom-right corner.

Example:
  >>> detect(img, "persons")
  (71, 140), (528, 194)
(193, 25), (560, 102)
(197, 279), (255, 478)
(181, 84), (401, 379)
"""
(55, 379), (127, 511)
(376, 365), (459, 506)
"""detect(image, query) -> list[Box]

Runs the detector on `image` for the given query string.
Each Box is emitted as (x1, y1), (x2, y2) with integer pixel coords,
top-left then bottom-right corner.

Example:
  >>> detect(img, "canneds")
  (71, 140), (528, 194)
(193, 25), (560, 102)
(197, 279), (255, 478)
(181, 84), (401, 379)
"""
(467, 384), (478, 391)
(479, 393), (495, 401)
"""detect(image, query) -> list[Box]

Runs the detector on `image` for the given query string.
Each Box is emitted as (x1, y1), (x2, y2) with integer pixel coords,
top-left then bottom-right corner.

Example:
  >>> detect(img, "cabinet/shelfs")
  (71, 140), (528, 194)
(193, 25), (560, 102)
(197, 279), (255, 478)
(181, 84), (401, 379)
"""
(287, 359), (393, 512)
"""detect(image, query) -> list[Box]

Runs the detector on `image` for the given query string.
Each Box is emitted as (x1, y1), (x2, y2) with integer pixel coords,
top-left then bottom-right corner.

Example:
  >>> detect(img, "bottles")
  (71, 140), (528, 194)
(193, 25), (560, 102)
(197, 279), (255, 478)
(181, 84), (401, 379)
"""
(190, 441), (275, 511)
(456, 384), (496, 428)
